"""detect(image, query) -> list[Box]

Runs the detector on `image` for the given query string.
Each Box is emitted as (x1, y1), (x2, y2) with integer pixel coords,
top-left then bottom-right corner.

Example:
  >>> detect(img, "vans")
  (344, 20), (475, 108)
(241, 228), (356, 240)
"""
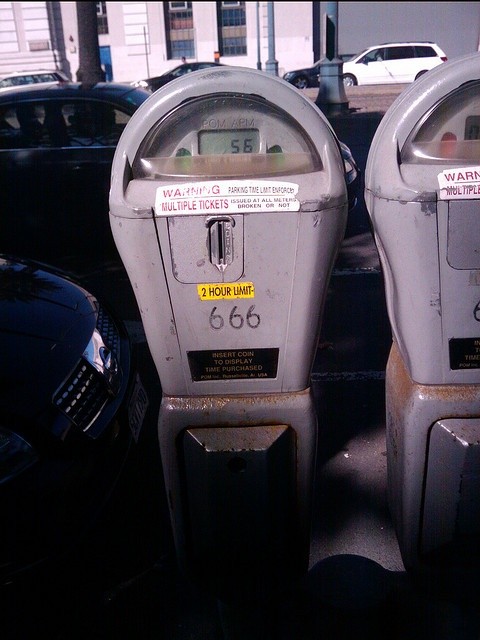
(1, 71), (69, 93)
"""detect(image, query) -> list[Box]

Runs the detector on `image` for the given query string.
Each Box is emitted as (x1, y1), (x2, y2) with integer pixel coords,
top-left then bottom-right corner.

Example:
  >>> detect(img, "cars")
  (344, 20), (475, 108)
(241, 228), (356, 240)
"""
(1, 260), (151, 507)
(283, 55), (356, 90)
(2, 89), (357, 276)
(128, 63), (229, 96)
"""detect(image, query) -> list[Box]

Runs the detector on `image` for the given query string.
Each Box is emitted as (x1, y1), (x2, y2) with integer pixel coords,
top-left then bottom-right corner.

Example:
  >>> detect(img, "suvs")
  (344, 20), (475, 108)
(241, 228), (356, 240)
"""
(337, 42), (448, 85)
